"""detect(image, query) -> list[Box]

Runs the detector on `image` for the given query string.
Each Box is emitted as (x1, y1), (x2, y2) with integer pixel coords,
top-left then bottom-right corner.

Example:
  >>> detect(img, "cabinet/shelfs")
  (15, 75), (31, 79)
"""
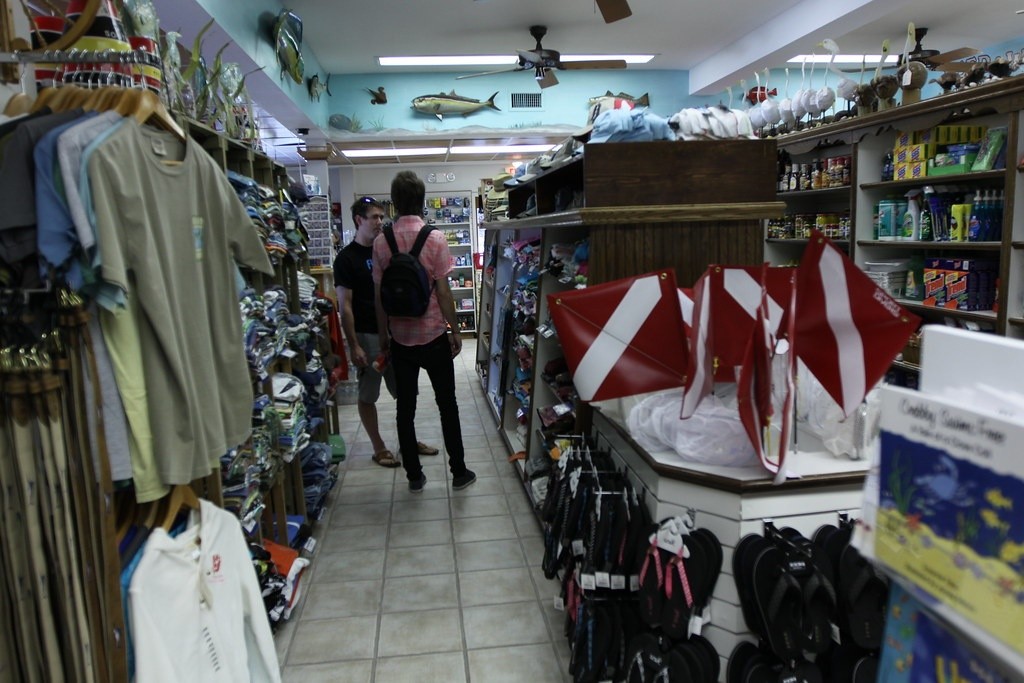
(166, 74), (1024, 553)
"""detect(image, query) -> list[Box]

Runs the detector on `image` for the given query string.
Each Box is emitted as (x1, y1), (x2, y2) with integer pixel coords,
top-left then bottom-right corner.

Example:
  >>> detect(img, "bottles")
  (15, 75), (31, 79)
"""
(762, 154), (851, 241)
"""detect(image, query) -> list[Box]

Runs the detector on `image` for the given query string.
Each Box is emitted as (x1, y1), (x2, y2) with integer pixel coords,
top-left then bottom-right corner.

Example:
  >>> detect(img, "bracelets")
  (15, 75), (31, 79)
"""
(451, 327), (462, 334)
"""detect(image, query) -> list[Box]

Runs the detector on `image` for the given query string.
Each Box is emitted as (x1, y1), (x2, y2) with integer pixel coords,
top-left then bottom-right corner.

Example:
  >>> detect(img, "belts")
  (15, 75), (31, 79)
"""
(1, 288), (131, 683)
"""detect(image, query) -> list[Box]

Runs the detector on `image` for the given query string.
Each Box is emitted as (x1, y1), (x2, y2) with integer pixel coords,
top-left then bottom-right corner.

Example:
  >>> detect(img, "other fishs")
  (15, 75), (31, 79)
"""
(310, 72), (332, 103)
(272, 8), (304, 86)
(410, 89), (501, 122)
(366, 86), (387, 105)
(589, 90), (650, 107)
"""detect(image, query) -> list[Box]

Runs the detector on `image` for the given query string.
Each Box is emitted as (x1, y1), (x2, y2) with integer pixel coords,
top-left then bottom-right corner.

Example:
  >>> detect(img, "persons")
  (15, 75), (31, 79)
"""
(370, 169), (477, 492)
(337, 196), (439, 468)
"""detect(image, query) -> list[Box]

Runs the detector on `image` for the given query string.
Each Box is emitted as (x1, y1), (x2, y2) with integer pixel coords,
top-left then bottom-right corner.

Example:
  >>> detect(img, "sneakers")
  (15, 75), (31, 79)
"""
(452, 470), (477, 489)
(408, 474), (425, 493)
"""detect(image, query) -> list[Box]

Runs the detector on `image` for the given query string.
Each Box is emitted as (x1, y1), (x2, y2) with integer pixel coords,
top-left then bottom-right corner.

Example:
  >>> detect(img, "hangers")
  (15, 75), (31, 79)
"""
(1, 45), (188, 168)
(114, 478), (163, 572)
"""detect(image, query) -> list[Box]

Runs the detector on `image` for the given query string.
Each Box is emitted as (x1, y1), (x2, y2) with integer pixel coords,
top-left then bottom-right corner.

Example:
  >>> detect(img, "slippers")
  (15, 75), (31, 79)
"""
(542, 432), (720, 681)
(417, 441), (438, 456)
(723, 523), (885, 683)
(371, 451), (401, 468)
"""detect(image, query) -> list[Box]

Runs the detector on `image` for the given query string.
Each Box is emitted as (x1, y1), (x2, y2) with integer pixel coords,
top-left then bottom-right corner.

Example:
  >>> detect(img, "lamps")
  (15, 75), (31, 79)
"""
(518, 40), (566, 80)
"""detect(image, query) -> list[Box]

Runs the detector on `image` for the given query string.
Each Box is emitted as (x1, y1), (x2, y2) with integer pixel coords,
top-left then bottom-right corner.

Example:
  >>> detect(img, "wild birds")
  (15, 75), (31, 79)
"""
(726, 21), (1024, 139)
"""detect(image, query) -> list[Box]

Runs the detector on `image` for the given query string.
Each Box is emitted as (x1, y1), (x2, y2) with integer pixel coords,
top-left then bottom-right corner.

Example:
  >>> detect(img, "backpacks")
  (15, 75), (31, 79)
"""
(380, 222), (438, 317)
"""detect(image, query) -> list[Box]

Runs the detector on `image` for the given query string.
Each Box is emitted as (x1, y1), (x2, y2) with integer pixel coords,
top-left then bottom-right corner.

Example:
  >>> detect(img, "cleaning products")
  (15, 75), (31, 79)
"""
(869, 118), (1011, 316)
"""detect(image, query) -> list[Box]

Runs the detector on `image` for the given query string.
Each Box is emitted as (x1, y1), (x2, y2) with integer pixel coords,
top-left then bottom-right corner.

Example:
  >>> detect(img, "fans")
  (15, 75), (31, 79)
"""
(838, 27), (1013, 77)
(456, 24), (628, 89)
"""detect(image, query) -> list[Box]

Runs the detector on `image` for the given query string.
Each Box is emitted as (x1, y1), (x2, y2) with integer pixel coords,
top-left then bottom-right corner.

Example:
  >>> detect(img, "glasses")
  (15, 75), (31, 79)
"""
(361, 197), (376, 204)
